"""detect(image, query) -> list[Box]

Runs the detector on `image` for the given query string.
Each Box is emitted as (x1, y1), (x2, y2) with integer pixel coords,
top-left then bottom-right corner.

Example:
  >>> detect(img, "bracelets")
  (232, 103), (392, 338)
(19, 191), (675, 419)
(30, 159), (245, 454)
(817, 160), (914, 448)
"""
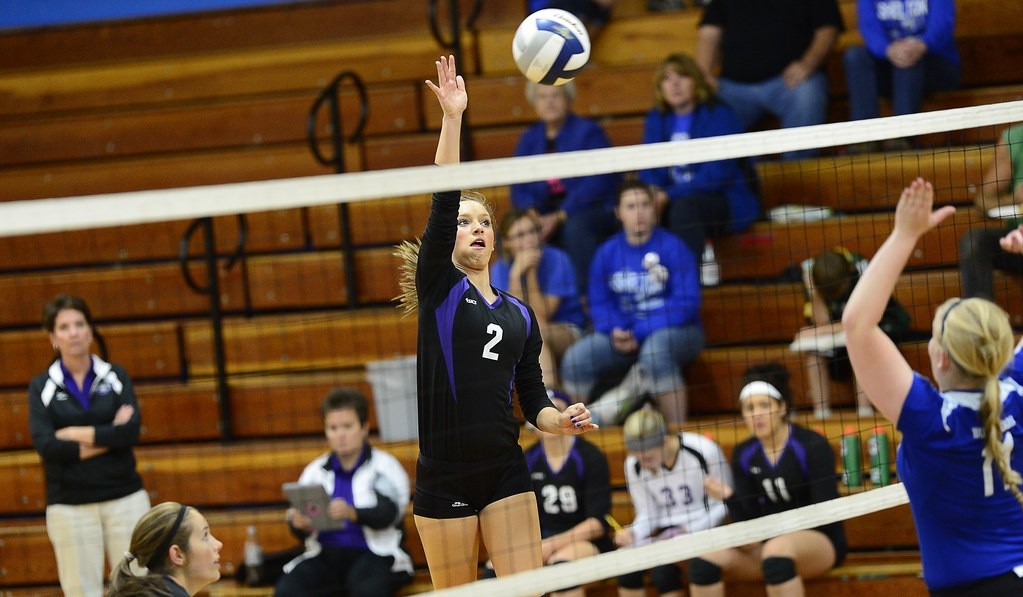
(555, 211), (565, 223)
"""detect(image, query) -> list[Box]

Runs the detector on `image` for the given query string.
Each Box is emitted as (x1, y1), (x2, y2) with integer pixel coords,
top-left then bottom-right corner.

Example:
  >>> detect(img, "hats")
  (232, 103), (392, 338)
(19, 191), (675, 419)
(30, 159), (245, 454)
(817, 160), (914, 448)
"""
(623, 403), (666, 450)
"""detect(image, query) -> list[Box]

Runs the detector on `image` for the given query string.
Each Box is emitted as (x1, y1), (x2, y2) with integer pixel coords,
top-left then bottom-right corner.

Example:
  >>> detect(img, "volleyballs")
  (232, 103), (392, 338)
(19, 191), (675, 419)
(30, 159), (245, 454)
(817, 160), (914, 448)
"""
(512, 9), (591, 87)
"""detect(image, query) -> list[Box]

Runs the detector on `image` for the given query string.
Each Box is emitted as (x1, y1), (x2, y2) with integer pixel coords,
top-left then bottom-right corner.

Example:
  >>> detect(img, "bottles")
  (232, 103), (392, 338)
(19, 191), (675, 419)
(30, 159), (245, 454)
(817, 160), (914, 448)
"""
(699, 237), (720, 288)
(840, 425), (862, 487)
(243, 527), (263, 587)
(867, 424), (890, 485)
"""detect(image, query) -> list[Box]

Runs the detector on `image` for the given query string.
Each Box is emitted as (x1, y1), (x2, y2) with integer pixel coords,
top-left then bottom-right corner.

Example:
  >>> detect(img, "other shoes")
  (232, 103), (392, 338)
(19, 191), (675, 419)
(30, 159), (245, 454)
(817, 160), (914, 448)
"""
(848, 142), (876, 155)
(883, 139), (909, 151)
(814, 406), (834, 421)
(857, 405), (874, 419)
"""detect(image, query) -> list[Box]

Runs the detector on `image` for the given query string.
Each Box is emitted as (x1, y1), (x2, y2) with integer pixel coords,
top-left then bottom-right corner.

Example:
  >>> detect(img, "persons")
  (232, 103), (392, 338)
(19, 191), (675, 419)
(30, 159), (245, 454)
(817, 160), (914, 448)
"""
(844, 0), (963, 153)
(795, 246), (912, 419)
(562, 184), (703, 426)
(615, 392), (735, 597)
(106, 502), (224, 597)
(842, 177), (1023, 597)
(636, 50), (764, 287)
(959, 124), (1023, 308)
(486, 208), (588, 428)
(274, 387), (415, 597)
(483, 386), (613, 597)
(28, 294), (152, 597)
(696, 0), (847, 160)
(689, 362), (847, 597)
(413, 55), (599, 597)
(509, 72), (622, 291)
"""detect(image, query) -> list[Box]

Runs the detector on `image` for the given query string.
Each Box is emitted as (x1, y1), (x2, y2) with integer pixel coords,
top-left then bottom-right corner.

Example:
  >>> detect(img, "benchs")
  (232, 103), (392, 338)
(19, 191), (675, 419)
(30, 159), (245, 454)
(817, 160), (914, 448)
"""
(0, 0), (1023, 597)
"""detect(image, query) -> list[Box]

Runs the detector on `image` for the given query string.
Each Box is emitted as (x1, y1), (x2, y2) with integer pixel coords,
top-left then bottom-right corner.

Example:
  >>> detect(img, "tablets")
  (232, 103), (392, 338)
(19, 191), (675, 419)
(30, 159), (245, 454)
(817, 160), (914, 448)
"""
(283, 483), (347, 532)
